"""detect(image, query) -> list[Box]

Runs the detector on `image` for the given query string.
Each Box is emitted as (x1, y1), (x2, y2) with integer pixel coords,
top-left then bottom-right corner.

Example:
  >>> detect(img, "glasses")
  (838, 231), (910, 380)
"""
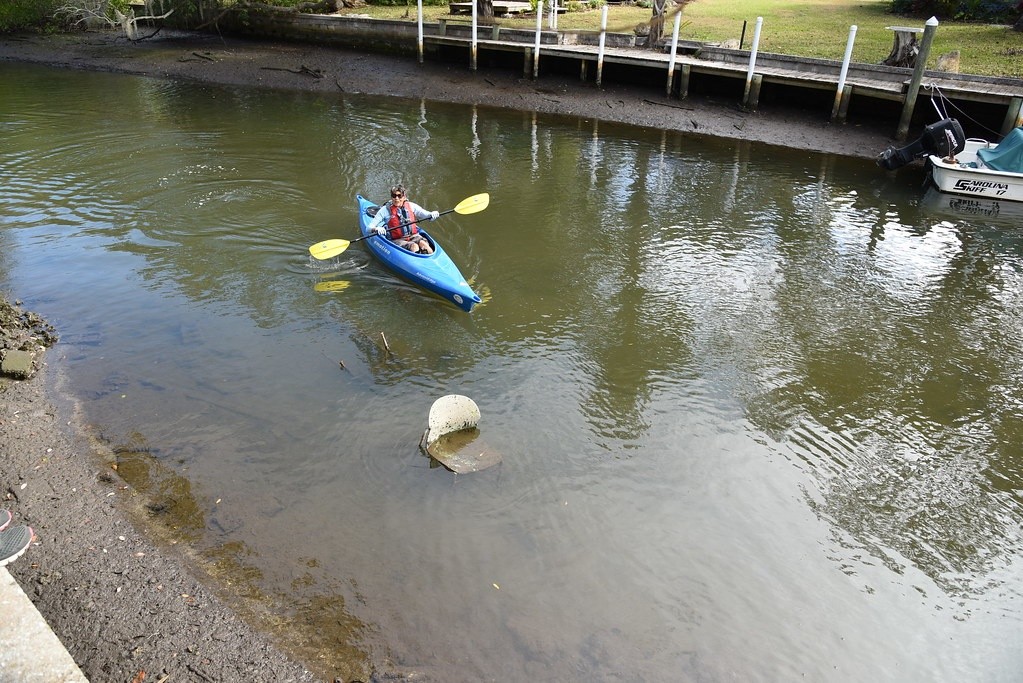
(391, 194), (403, 198)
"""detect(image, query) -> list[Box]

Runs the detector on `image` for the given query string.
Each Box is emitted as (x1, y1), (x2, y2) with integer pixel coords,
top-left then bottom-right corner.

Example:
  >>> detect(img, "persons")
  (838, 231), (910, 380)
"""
(367, 185), (440, 253)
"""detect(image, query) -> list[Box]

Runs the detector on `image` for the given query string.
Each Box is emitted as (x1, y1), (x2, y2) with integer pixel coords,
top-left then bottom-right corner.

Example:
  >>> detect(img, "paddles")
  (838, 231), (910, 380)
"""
(308, 192), (490, 261)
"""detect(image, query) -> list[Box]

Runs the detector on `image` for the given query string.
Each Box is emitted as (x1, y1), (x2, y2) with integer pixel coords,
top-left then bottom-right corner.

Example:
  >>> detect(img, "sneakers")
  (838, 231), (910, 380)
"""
(0, 525), (33, 567)
(0, 509), (12, 532)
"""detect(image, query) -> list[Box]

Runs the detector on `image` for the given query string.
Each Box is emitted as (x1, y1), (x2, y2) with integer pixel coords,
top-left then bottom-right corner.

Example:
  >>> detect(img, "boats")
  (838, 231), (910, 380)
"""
(356, 194), (482, 313)
(875, 117), (1023, 201)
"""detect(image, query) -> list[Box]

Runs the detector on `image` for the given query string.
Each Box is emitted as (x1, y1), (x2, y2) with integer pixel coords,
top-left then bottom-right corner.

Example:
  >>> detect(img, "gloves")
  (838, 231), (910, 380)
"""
(375, 227), (386, 236)
(429, 211), (439, 219)
